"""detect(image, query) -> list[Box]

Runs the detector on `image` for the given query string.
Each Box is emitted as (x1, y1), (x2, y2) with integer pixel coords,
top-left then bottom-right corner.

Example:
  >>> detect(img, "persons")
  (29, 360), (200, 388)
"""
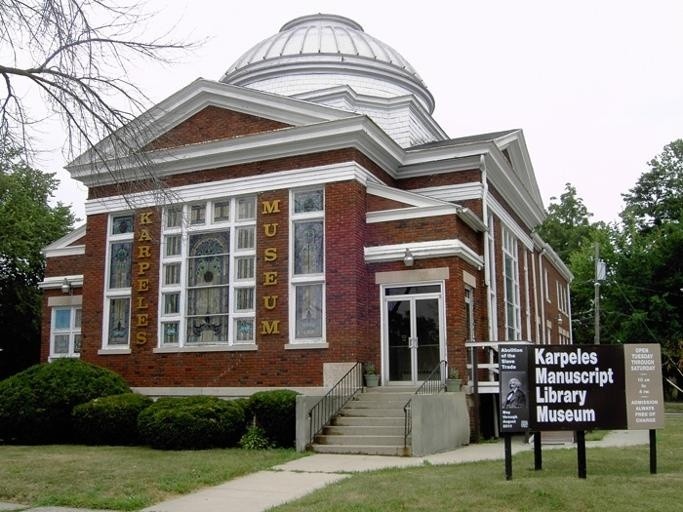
(505, 377), (526, 408)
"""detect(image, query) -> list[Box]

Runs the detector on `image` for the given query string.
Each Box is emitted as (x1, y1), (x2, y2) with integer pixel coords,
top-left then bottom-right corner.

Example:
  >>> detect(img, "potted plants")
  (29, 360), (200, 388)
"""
(446, 365), (463, 392)
(363, 364), (380, 386)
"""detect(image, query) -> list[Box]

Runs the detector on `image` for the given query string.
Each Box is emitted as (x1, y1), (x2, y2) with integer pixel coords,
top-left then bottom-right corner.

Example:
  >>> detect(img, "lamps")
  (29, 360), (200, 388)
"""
(403, 247), (414, 267)
(553, 313), (563, 324)
(61, 278), (73, 295)
